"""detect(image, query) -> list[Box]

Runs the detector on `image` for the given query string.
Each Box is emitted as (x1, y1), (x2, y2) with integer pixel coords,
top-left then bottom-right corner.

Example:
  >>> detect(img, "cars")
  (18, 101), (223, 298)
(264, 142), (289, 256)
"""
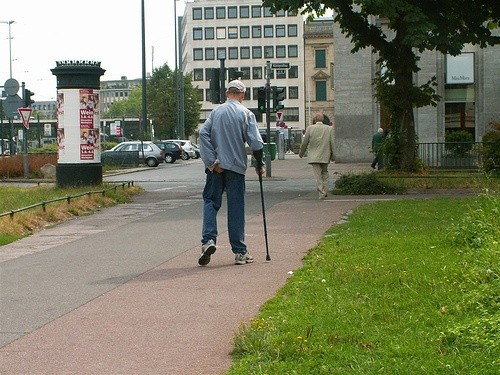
(441, 145), (469, 157)
(104, 140), (201, 168)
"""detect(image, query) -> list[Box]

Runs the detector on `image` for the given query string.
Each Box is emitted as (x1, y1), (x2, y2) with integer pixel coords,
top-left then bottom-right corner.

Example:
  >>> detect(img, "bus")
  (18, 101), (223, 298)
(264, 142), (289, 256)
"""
(99, 118), (156, 141)
(0, 119), (59, 156)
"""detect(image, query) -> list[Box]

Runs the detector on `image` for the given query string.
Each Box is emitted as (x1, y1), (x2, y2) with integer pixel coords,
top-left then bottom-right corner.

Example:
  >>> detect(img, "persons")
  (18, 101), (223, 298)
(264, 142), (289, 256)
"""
(199, 81), (267, 265)
(370, 128), (383, 171)
(299, 113), (335, 200)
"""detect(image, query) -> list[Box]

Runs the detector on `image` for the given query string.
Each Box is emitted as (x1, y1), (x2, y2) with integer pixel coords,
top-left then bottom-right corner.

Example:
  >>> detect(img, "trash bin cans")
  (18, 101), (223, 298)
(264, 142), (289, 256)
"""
(262, 143), (276, 161)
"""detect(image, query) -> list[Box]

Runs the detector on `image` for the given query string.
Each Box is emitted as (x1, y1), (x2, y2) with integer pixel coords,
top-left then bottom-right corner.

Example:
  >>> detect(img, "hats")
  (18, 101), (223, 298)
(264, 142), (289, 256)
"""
(227, 80), (246, 92)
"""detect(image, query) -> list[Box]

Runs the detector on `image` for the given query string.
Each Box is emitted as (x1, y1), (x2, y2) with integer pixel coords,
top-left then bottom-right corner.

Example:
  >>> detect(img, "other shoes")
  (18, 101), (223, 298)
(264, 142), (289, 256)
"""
(371, 166), (376, 169)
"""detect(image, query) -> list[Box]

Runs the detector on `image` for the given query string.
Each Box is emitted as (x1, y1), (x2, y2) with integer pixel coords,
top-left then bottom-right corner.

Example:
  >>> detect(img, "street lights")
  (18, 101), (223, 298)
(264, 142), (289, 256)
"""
(2, 21), (16, 79)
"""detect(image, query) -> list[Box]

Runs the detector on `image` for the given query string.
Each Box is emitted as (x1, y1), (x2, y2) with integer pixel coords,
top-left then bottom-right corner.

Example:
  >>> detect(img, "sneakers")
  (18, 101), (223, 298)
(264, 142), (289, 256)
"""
(198, 239), (216, 265)
(235, 252), (253, 265)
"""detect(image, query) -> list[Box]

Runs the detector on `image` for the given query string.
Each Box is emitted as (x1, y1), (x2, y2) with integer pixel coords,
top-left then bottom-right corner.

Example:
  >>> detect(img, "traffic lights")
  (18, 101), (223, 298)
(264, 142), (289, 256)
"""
(257, 87), (267, 112)
(25, 89), (35, 107)
(273, 86), (284, 113)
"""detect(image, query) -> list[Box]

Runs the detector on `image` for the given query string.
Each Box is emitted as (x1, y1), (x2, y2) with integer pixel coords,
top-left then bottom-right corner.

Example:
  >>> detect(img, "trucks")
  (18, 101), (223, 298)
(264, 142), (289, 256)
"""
(258, 128), (277, 142)
